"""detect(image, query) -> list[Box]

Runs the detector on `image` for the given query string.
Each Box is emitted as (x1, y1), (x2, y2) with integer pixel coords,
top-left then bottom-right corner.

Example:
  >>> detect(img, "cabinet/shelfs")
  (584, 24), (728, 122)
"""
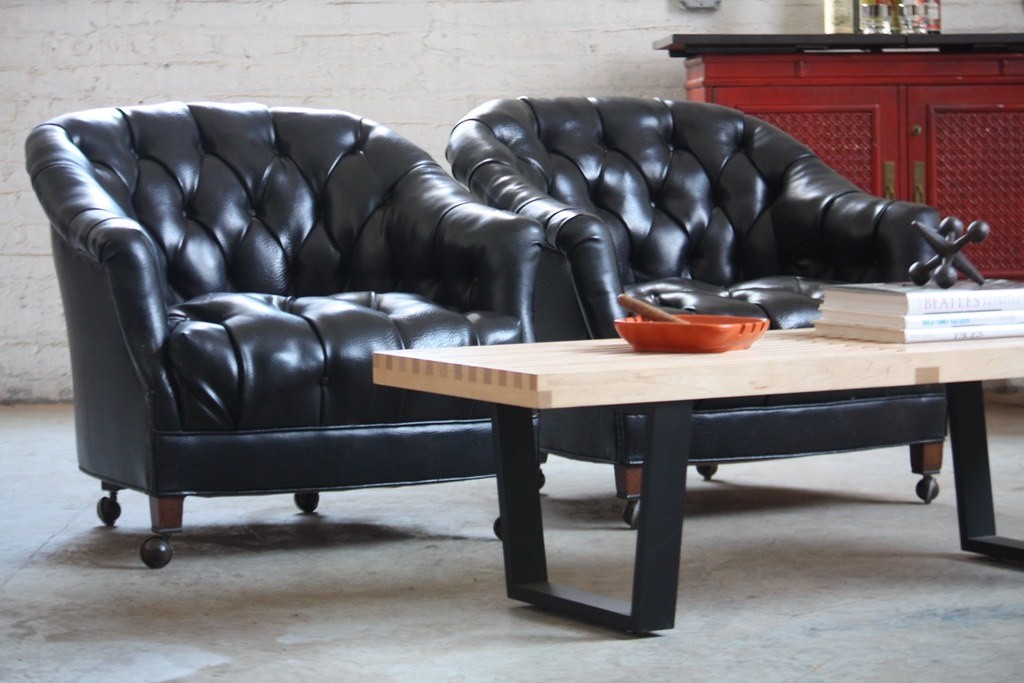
(652, 32), (1024, 282)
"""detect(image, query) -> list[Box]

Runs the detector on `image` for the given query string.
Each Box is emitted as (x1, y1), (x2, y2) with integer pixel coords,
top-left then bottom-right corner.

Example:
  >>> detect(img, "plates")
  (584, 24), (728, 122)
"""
(612, 315), (770, 354)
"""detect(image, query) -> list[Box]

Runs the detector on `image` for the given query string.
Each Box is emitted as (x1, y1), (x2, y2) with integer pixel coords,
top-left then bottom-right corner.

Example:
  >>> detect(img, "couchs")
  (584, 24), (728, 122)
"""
(446, 96), (949, 529)
(24, 101), (547, 570)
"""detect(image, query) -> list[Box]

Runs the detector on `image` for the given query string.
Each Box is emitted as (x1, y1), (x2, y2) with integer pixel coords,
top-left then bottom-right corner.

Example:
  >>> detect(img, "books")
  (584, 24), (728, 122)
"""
(812, 283), (1024, 344)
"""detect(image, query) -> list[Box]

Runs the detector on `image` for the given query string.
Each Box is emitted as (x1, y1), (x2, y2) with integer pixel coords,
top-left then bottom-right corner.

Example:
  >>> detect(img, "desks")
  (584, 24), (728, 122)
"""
(372, 335), (1024, 634)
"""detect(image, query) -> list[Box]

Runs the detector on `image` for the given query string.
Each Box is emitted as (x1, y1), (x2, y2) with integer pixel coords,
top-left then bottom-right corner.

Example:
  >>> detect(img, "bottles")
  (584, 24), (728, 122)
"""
(823, 0), (941, 35)
